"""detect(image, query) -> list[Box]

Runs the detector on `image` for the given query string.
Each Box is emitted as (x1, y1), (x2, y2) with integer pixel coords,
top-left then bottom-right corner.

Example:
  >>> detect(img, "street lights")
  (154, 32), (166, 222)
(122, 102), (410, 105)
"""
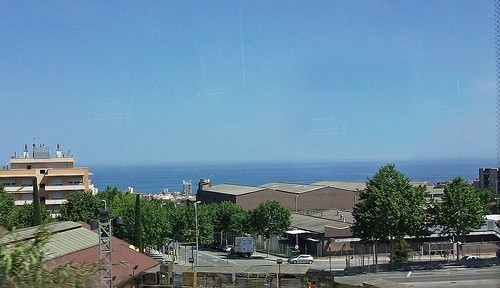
(277, 259), (282, 288)
(194, 200), (202, 266)
(189, 258), (194, 271)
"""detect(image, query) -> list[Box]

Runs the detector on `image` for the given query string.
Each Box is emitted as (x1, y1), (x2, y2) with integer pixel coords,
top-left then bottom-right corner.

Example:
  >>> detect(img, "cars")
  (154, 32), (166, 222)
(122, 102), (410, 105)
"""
(287, 254), (314, 264)
(223, 245), (232, 252)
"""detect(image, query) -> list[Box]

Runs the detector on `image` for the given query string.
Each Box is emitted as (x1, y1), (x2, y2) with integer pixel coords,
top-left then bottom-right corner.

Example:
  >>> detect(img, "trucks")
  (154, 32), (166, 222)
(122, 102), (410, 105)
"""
(234, 236), (254, 257)
